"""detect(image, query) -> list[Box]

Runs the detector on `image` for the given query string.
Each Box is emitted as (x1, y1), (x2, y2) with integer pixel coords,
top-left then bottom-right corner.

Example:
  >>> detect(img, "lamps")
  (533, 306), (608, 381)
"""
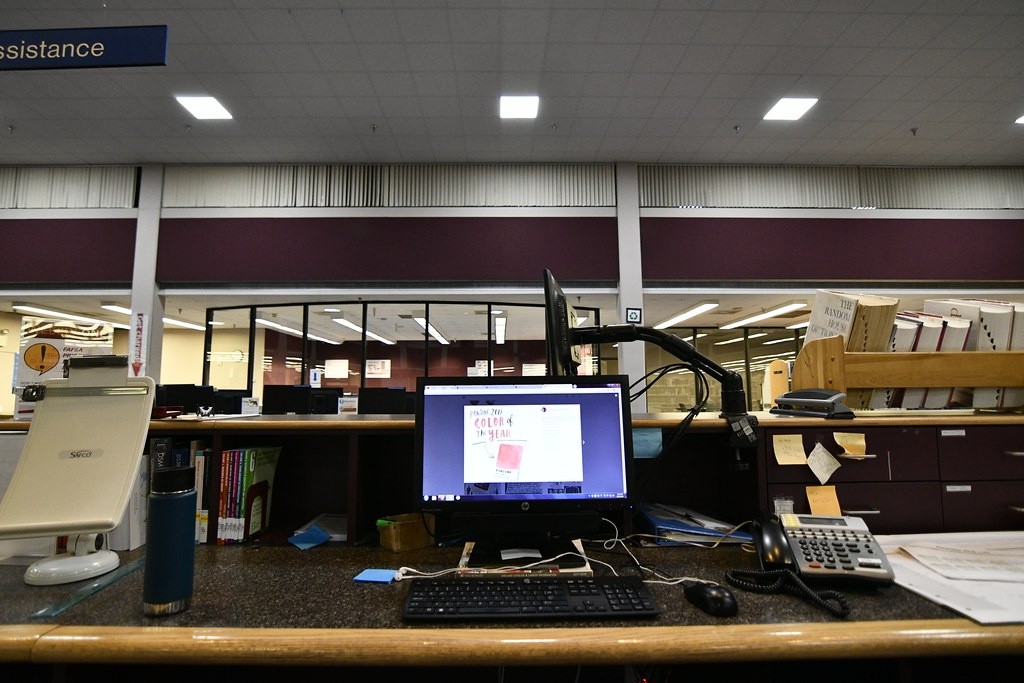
(255, 311), (343, 344)
(612, 300), (719, 347)
(102, 302), (206, 331)
(331, 312), (396, 344)
(575, 310), (588, 326)
(495, 310), (507, 345)
(11, 301), (130, 330)
(412, 310), (451, 345)
(719, 301), (808, 330)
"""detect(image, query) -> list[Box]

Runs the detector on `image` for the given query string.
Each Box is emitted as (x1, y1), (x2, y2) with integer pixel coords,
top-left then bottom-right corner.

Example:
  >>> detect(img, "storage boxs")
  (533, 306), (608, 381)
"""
(377, 510), (438, 554)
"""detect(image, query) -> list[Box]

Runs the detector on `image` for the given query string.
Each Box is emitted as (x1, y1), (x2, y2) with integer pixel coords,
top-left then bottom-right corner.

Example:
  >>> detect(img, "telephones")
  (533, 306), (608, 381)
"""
(758, 512), (894, 586)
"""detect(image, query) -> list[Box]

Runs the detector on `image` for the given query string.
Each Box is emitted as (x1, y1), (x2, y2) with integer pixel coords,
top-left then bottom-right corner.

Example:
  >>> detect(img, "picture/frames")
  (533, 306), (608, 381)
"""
(626, 308), (642, 323)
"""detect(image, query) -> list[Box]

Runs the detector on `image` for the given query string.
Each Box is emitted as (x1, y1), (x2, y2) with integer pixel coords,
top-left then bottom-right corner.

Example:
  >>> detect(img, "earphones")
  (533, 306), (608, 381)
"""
(396, 573), (413, 580)
(400, 567), (421, 574)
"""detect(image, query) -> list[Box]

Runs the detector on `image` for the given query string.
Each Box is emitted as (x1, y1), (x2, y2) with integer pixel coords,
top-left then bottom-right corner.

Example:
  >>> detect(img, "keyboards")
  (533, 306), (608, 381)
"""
(401, 574), (660, 623)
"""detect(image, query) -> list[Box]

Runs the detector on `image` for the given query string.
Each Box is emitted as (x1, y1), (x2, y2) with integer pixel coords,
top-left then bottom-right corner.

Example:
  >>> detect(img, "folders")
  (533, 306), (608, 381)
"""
(638, 500), (754, 547)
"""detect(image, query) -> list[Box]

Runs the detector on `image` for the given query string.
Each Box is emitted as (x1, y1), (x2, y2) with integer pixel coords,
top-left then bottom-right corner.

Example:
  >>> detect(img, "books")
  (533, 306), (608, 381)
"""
(455, 540), (593, 577)
(108, 437), (279, 551)
(802, 288), (1024, 410)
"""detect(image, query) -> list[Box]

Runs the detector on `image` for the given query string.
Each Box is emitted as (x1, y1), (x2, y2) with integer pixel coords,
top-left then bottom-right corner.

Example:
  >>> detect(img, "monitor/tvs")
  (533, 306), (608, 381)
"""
(415, 375), (636, 572)
(156, 384), (416, 415)
(542, 268), (582, 376)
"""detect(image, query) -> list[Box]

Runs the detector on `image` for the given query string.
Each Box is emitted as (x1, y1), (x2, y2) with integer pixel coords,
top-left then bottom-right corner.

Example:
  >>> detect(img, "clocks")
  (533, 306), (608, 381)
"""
(232, 350), (244, 361)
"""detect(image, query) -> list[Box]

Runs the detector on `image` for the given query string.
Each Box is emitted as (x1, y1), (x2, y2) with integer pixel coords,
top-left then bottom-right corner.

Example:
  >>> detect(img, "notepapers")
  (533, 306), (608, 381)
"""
(353, 569), (397, 584)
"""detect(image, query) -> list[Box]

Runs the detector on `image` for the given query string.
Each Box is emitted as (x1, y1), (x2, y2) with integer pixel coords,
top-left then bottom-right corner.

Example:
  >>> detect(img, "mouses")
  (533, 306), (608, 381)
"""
(688, 582), (738, 618)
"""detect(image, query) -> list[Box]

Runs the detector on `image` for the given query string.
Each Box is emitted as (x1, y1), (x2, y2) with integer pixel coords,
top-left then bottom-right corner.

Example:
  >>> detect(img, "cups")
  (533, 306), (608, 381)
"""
(145, 466), (197, 615)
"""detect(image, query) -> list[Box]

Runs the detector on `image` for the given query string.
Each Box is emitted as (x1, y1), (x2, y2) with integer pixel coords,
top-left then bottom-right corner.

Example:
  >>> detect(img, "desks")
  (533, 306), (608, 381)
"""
(0, 530), (1024, 683)
